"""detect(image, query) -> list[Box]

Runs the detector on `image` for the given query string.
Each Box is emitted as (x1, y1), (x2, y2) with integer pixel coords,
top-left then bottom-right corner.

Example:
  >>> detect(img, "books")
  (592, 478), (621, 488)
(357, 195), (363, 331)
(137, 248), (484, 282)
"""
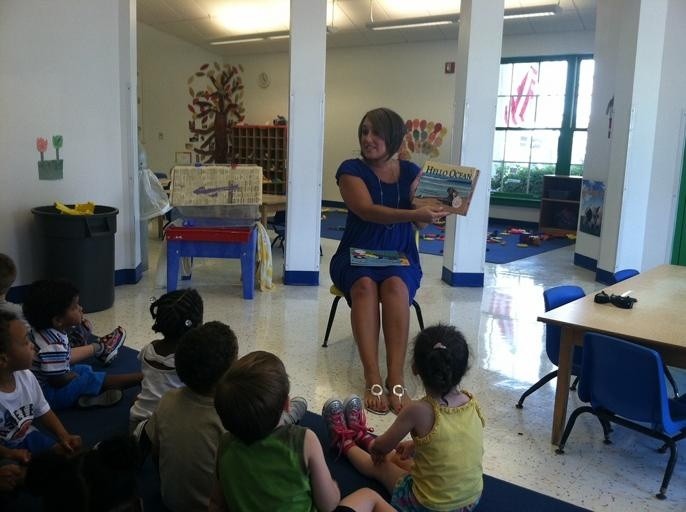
(349, 247), (410, 267)
(411, 159), (480, 216)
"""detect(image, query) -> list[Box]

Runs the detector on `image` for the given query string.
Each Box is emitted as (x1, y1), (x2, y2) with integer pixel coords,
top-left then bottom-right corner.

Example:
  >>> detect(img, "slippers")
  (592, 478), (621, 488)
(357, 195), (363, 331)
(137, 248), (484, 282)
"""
(364, 384), (391, 415)
(385, 383), (413, 416)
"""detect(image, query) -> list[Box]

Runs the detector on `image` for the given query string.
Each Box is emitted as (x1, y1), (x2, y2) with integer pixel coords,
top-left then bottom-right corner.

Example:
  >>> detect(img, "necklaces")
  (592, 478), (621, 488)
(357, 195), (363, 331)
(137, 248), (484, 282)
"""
(369, 159), (401, 231)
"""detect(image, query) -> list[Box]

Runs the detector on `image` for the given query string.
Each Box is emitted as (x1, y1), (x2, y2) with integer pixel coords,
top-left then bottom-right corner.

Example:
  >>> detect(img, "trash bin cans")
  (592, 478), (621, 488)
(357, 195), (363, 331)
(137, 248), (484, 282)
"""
(31, 204), (120, 314)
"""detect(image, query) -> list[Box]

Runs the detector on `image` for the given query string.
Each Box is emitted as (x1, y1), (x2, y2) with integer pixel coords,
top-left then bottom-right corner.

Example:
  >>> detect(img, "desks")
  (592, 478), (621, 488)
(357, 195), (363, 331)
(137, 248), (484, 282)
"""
(152, 193), (287, 241)
(537, 263), (686, 445)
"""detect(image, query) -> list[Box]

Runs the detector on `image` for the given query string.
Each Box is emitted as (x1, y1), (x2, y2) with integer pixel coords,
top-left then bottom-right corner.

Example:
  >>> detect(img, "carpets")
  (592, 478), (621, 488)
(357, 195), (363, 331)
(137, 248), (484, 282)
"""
(267, 210), (576, 265)
(14, 334), (595, 512)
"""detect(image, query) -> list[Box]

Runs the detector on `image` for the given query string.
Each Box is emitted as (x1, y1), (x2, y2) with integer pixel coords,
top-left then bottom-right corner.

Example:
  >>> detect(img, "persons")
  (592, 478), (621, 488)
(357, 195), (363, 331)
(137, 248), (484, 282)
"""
(21, 277), (144, 411)
(0, 253), (126, 367)
(0, 310), (83, 494)
(128, 320), (308, 512)
(127, 289), (203, 449)
(207, 351), (400, 512)
(329, 108), (452, 415)
(322, 326), (487, 512)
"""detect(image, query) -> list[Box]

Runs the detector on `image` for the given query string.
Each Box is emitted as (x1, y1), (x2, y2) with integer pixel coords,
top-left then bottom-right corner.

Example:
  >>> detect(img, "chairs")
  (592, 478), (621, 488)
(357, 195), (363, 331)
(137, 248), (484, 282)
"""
(321, 227), (424, 348)
(271, 224), (285, 251)
(164, 163), (263, 300)
(610, 269), (640, 285)
(554, 330), (686, 499)
(514, 285), (616, 435)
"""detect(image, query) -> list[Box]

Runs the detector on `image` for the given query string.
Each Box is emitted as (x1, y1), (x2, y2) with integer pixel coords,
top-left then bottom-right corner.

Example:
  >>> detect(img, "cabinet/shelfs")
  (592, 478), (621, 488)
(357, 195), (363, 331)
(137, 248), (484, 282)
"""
(538, 175), (582, 242)
(231, 126), (286, 193)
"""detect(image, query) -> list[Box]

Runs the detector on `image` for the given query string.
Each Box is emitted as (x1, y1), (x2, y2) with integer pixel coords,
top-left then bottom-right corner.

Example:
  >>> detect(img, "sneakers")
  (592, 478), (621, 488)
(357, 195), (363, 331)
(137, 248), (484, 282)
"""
(78, 388), (123, 409)
(69, 320), (92, 349)
(284, 396), (308, 426)
(322, 398), (356, 464)
(93, 326), (126, 369)
(343, 394), (373, 444)
